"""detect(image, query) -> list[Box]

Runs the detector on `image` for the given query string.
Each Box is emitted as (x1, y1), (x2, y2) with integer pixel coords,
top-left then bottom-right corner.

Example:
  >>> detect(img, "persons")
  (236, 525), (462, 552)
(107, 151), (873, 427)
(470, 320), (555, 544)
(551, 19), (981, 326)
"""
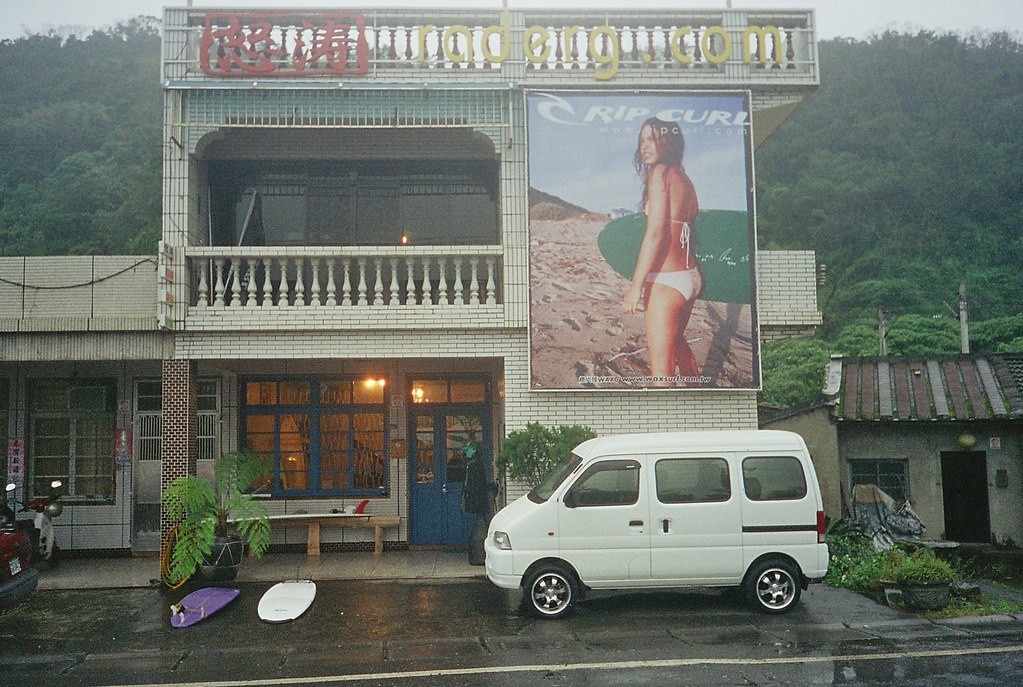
(621, 116), (703, 388)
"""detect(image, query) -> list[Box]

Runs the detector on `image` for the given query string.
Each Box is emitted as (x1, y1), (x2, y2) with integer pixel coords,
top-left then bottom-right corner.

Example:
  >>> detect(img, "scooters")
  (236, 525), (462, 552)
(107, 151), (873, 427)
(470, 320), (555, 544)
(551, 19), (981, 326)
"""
(0, 479), (63, 585)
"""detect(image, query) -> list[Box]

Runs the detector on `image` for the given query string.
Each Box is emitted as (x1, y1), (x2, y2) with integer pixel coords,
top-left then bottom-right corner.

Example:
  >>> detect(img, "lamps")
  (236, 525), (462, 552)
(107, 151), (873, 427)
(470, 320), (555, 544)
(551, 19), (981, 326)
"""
(956, 430), (976, 449)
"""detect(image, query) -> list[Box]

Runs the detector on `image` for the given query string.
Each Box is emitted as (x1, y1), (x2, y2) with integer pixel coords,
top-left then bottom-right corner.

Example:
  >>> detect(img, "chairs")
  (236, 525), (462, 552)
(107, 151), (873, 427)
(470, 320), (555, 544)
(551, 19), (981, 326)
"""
(745, 477), (763, 500)
(704, 469), (729, 501)
(655, 469), (670, 494)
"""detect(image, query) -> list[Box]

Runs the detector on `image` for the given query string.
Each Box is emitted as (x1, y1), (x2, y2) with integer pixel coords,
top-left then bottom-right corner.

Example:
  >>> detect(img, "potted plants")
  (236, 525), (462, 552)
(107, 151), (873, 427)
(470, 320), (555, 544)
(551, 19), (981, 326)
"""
(880, 544), (956, 609)
(163, 446), (280, 590)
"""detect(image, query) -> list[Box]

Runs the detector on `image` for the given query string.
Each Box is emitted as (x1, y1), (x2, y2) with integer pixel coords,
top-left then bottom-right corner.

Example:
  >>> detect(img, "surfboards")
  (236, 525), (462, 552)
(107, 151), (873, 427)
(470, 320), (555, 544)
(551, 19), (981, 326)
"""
(596, 208), (752, 305)
(170, 586), (241, 629)
(257, 579), (317, 625)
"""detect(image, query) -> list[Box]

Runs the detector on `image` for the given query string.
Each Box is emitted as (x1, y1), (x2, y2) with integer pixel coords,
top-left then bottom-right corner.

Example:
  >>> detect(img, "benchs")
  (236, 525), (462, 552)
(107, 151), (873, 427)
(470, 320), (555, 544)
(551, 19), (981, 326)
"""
(247, 514), (401, 560)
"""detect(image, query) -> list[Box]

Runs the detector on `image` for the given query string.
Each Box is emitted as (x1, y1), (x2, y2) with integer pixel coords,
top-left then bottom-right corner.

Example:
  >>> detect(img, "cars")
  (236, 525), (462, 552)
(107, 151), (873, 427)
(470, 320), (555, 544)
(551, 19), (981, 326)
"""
(0, 494), (40, 612)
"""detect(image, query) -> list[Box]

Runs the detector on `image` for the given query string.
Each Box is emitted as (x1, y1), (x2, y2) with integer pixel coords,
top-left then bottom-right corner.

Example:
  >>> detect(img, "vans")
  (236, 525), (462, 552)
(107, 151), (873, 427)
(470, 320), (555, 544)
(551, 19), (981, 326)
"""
(484, 428), (832, 613)
